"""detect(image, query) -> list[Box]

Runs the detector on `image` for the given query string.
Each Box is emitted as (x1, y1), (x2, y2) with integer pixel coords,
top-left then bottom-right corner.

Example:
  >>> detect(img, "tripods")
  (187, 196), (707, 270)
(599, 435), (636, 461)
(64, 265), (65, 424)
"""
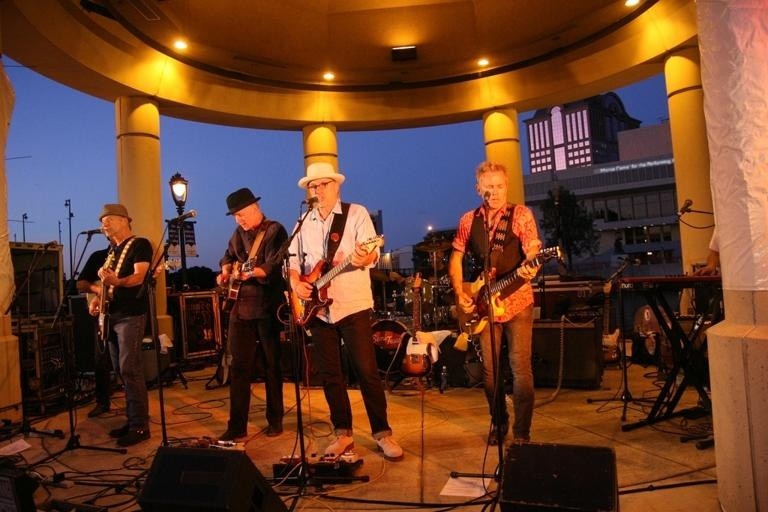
(262, 205), (369, 512)
(0, 248), (66, 442)
(19, 234), (128, 472)
(587, 266), (672, 421)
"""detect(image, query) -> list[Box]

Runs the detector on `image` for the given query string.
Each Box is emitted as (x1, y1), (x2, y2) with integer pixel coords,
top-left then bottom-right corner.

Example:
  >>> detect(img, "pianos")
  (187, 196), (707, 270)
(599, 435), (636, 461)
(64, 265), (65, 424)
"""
(621, 275), (721, 291)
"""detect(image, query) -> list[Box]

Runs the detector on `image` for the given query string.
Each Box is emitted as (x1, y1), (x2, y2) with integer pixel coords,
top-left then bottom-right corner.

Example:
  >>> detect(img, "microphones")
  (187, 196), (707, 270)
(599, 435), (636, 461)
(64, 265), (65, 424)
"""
(40, 240), (57, 248)
(169, 209), (197, 224)
(484, 189), (492, 200)
(678, 199), (692, 217)
(80, 227), (105, 234)
(302, 195), (320, 205)
(618, 256), (641, 266)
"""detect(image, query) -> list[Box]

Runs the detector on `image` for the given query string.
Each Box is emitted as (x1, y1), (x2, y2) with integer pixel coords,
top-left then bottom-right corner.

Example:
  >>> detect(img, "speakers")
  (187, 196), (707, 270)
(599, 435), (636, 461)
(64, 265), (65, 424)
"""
(499, 440), (619, 512)
(138, 446), (289, 512)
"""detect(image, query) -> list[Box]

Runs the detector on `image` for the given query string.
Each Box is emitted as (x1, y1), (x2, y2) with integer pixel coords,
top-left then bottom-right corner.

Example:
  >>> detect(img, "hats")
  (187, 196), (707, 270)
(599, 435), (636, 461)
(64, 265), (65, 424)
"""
(297, 162), (346, 190)
(98, 203), (132, 226)
(224, 188), (261, 216)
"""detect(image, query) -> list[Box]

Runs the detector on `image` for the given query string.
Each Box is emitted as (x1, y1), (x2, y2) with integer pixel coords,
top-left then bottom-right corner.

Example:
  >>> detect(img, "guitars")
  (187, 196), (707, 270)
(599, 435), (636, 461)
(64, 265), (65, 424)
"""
(284, 234), (385, 326)
(222, 256), (257, 312)
(454, 247), (560, 336)
(601, 277), (621, 365)
(97, 254), (115, 340)
(403, 274), (431, 375)
(86, 259), (181, 308)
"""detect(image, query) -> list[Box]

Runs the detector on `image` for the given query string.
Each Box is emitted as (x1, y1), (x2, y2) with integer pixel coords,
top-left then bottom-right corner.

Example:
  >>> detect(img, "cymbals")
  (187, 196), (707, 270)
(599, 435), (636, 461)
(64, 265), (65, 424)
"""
(415, 240), (451, 253)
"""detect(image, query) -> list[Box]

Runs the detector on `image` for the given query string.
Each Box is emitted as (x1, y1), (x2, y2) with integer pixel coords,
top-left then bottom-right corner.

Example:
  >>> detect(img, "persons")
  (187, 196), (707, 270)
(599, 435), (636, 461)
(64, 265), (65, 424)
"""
(693, 225), (721, 275)
(448, 161), (542, 447)
(215, 188), (288, 441)
(286, 162), (403, 463)
(76, 235), (122, 418)
(98, 204), (152, 447)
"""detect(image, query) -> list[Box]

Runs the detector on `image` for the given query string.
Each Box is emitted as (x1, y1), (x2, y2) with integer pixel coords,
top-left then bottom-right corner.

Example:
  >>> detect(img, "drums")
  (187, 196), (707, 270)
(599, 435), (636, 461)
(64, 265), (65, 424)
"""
(370, 317), (414, 376)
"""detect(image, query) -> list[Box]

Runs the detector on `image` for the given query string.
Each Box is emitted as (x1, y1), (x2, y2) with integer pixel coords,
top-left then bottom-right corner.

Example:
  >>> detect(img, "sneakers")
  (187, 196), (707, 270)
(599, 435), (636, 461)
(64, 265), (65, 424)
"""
(267, 421), (282, 436)
(117, 430), (151, 445)
(325, 435), (355, 459)
(375, 436), (404, 462)
(219, 430), (247, 440)
(87, 406), (110, 417)
(487, 423), (508, 445)
(109, 425), (130, 438)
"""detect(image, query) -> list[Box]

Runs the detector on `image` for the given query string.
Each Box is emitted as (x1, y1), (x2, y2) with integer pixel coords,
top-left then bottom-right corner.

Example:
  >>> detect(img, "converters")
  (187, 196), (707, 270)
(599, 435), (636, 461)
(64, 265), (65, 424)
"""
(54, 473), (66, 484)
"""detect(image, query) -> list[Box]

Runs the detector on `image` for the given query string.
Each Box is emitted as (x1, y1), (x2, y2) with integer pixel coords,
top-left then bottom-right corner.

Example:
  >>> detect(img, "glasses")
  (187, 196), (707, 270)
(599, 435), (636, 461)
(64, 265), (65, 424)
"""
(308, 181), (333, 189)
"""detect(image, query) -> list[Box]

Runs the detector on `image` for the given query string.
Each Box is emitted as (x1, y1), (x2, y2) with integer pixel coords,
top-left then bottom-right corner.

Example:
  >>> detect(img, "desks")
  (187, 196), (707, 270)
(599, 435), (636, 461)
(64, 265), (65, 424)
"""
(621, 273), (724, 432)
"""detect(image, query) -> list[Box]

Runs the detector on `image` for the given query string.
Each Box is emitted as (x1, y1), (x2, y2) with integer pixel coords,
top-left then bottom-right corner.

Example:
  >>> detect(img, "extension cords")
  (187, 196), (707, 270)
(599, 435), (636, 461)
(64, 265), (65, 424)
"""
(41, 477), (74, 489)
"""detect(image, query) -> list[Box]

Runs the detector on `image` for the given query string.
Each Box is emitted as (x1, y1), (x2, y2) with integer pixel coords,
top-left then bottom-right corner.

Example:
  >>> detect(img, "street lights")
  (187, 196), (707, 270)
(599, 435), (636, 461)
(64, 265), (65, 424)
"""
(58, 220), (63, 247)
(65, 198), (73, 279)
(22, 212), (27, 244)
(548, 170), (568, 275)
(170, 169), (189, 287)
(426, 225), (438, 275)
